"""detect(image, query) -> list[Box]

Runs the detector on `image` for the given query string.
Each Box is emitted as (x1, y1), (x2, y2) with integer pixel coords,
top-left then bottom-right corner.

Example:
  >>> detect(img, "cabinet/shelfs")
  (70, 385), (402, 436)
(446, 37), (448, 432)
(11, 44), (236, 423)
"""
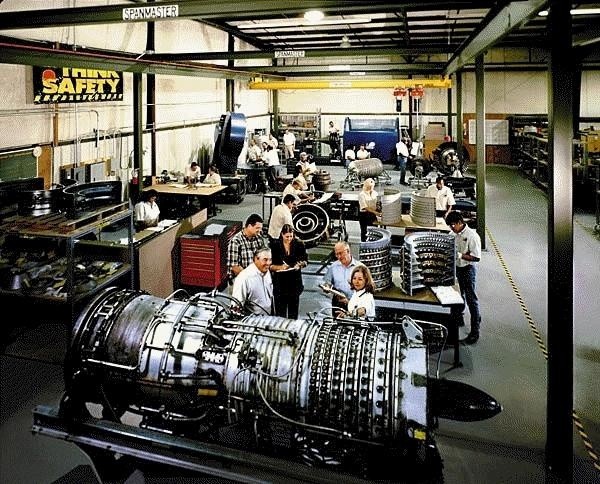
(277, 112), (321, 156)
(0, 195), (140, 366)
(506, 111), (588, 203)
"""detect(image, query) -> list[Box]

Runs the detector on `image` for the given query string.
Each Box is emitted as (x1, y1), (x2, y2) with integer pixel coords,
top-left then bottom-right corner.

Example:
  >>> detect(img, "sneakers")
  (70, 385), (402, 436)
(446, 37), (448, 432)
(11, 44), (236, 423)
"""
(466, 330), (480, 345)
(459, 319), (465, 327)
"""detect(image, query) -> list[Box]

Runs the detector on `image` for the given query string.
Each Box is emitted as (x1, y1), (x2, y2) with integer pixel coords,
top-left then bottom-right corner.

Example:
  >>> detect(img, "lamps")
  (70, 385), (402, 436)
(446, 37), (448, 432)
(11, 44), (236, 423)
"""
(338, 34), (354, 49)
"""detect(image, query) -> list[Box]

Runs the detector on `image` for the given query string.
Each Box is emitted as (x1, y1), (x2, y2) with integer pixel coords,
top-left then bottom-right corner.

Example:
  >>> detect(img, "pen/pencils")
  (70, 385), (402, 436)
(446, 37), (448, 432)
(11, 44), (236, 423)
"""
(282, 260), (289, 266)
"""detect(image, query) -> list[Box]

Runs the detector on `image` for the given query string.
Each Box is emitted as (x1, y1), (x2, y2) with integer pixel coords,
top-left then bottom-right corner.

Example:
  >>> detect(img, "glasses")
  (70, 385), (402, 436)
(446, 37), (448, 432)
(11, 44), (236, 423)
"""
(449, 223), (457, 228)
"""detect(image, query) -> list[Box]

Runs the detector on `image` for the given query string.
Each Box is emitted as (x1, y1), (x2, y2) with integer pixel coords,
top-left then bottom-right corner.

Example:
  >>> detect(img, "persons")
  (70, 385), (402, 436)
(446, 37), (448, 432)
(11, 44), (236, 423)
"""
(183, 161), (201, 185)
(203, 165), (221, 186)
(134, 189), (160, 233)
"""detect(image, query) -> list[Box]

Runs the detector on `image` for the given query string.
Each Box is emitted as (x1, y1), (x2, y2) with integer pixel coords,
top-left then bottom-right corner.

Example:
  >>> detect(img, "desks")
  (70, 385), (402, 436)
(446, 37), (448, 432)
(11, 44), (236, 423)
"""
(207, 173), (251, 205)
(371, 268), (468, 368)
(142, 180), (226, 217)
(259, 189), (284, 221)
(371, 211), (452, 244)
